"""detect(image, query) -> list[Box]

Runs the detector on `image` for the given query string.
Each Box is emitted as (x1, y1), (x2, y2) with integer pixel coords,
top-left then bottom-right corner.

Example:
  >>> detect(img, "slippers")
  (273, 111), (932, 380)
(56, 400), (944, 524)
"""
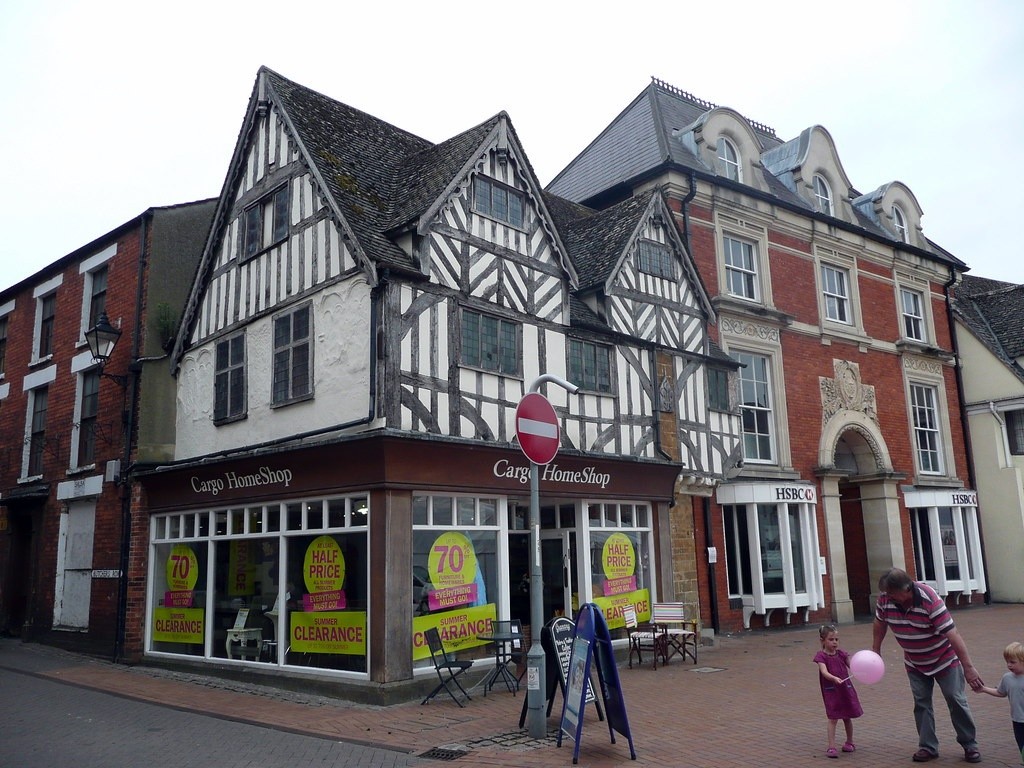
(842, 741), (856, 751)
(826, 747), (838, 757)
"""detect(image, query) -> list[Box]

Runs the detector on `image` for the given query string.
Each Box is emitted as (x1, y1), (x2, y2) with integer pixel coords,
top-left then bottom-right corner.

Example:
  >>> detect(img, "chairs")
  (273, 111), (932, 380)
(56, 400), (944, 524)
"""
(490, 619), (531, 691)
(622, 603), (667, 671)
(651, 602), (698, 667)
(420, 627), (474, 708)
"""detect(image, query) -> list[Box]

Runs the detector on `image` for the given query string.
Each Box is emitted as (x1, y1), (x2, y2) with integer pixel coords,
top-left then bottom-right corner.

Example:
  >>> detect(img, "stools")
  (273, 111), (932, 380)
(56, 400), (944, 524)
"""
(226, 627), (265, 665)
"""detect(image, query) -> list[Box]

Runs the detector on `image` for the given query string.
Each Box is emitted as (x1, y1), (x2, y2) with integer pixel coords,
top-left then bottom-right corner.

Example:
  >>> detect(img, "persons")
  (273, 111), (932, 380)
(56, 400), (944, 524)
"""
(971, 642), (1024, 766)
(872, 567), (985, 762)
(813, 624), (863, 758)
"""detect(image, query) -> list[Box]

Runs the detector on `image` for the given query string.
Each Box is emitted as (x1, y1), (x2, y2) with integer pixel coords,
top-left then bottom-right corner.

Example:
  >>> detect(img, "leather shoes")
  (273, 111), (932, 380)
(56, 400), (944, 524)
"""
(913, 749), (940, 761)
(964, 750), (982, 763)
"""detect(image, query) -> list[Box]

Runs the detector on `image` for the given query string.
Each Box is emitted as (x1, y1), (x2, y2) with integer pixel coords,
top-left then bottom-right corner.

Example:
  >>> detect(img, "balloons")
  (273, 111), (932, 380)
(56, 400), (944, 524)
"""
(850, 649), (885, 685)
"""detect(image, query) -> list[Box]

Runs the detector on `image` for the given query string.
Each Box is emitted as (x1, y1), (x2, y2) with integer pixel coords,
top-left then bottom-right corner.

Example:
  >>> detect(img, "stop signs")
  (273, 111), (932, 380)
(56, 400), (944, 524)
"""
(515, 390), (560, 465)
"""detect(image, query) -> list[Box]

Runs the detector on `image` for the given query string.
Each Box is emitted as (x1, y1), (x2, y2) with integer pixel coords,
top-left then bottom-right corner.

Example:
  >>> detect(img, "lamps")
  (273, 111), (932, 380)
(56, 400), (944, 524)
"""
(84, 308), (128, 391)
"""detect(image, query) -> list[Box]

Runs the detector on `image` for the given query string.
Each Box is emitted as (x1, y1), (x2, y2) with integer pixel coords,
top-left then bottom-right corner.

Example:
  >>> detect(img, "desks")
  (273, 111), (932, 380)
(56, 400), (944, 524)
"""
(477, 633), (525, 697)
